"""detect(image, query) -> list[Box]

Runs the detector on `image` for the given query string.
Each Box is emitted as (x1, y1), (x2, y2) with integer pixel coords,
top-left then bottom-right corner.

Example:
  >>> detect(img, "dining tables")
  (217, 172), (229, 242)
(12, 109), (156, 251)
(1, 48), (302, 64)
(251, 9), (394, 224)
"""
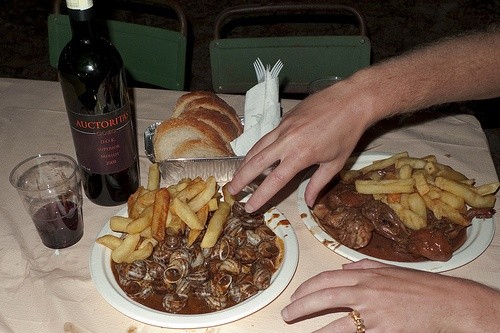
(0, 77), (500, 333)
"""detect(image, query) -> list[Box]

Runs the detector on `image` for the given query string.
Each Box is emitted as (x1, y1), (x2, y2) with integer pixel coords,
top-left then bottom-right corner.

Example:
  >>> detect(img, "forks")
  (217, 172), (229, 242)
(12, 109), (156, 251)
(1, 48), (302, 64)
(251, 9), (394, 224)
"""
(269, 58), (283, 77)
(252, 57), (266, 83)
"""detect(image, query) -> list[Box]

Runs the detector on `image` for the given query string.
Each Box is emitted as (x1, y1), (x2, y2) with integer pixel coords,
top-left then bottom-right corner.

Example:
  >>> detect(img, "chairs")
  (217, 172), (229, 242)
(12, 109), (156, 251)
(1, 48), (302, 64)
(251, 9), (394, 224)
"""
(209, 3), (371, 95)
(47, 0), (187, 92)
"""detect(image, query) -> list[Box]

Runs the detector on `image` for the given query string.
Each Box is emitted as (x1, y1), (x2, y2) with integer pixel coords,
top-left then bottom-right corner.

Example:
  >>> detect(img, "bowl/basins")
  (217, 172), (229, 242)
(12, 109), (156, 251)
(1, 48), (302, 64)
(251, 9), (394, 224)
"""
(145, 115), (246, 185)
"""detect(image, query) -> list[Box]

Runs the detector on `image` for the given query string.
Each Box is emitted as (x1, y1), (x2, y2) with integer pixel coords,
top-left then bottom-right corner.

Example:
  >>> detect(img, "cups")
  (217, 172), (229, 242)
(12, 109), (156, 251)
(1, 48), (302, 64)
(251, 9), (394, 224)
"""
(307, 76), (345, 97)
(9, 153), (85, 250)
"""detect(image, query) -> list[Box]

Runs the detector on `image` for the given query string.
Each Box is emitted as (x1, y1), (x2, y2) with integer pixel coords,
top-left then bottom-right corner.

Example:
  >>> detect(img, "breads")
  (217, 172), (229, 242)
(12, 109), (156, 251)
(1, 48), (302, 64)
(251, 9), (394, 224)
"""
(154, 91), (243, 180)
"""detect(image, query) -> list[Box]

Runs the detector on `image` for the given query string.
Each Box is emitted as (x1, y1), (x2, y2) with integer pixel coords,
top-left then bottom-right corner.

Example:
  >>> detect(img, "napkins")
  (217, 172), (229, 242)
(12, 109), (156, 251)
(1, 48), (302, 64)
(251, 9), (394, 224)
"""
(229, 79), (280, 157)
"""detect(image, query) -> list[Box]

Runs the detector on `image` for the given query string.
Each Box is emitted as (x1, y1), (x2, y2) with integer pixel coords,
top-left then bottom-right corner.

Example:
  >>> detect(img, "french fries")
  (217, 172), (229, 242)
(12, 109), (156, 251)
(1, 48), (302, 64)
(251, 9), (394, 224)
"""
(352, 152), (500, 230)
(94, 164), (235, 263)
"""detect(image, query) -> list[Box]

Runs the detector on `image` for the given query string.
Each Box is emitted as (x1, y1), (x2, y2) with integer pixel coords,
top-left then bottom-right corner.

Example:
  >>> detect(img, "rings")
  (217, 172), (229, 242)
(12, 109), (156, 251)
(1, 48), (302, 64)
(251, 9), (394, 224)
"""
(350, 311), (366, 333)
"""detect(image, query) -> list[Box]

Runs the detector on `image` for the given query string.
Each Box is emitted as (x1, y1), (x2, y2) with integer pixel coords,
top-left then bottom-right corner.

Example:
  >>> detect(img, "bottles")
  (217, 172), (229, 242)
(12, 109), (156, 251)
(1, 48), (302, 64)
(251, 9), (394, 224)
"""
(57, 0), (140, 207)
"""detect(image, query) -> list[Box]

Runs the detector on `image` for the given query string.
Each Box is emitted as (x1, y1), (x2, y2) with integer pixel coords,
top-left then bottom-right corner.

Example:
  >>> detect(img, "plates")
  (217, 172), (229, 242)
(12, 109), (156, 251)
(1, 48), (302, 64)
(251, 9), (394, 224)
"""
(297, 154), (494, 273)
(89, 187), (298, 329)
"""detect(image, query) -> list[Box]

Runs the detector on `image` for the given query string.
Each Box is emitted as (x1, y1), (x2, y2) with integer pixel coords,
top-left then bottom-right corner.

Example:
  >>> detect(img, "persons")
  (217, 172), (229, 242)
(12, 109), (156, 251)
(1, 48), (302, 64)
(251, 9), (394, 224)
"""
(227, 25), (500, 333)
